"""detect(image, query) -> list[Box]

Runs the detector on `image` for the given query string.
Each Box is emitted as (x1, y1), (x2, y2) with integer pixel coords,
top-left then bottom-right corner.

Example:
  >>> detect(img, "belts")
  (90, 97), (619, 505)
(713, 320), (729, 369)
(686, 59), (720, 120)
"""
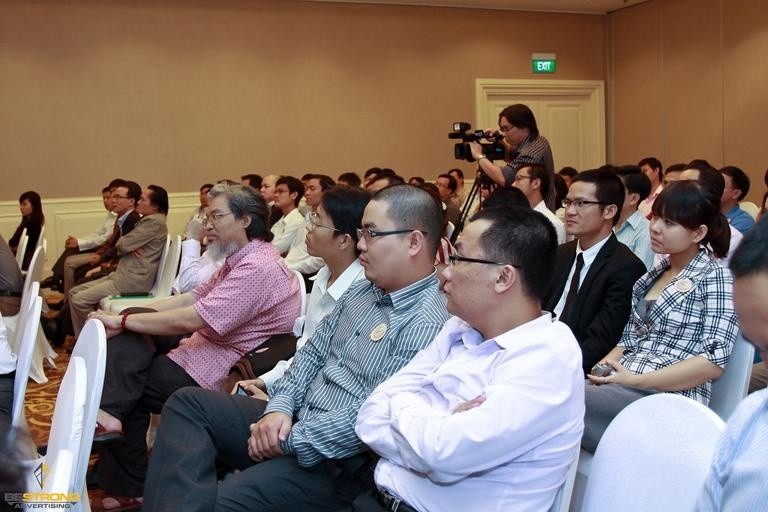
(0, 289), (21, 297)
(372, 485), (415, 511)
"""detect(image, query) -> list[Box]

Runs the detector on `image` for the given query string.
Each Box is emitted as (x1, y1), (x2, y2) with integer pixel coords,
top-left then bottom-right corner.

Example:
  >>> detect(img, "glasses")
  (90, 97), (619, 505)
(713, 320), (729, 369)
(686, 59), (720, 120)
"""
(304, 211), (346, 233)
(499, 124), (514, 132)
(355, 227), (430, 243)
(201, 212), (233, 226)
(113, 194), (131, 200)
(449, 249), (523, 270)
(513, 174), (537, 182)
(273, 190), (289, 194)
(561, 198), (614, 209)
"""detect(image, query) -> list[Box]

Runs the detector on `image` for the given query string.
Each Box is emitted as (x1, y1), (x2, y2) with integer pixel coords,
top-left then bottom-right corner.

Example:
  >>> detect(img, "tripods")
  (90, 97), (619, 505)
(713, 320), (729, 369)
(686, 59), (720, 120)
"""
(445, 169), (502, 245)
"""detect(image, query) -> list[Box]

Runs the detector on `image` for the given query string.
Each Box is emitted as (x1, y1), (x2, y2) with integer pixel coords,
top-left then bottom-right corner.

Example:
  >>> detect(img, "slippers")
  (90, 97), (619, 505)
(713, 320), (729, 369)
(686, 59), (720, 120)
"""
(37, 419), (125, 456)
(90, 493), (143, 511)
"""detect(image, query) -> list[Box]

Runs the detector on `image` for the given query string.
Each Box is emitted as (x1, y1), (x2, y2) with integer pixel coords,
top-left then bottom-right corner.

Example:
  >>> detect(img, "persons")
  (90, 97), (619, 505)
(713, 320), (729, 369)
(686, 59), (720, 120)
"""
(9, 191), (43, 272)
(0, 233), (24, 316)
(36, 103), (768, 511)
(0, 319), (17, 421)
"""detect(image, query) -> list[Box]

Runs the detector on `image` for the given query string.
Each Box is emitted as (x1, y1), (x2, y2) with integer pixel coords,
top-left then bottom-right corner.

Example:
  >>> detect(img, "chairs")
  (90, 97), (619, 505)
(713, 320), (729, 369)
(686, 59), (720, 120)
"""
(572, 331), (759, 512)
(555, 463), (579, 512)
(282, 271), (310, 319)
(579, 390), (724, 511)
(2, 226), (120, 510)
(738, 200), (762, 226)
(102, 236), (191, 320)
(157, 230), (170, 277)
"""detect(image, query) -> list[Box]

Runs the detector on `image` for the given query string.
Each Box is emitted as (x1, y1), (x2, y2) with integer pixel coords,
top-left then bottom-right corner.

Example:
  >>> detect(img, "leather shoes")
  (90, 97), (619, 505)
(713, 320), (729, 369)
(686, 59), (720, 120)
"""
(39, 276), (75, 353)
